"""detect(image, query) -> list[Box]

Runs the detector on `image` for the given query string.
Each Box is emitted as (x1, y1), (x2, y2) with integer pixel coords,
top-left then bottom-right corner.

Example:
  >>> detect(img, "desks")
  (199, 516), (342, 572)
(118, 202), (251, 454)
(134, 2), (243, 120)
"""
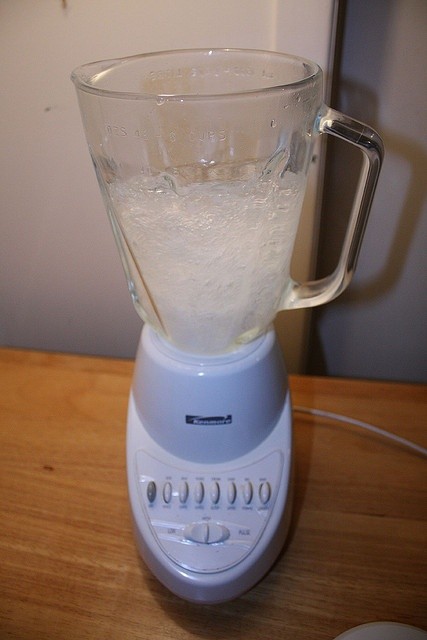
(3, 345), (427, 638)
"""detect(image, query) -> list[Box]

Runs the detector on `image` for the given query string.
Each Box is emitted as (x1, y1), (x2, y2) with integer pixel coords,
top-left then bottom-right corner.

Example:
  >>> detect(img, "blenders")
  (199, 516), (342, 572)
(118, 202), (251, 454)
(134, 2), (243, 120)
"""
(67, 43), (386, 608)
